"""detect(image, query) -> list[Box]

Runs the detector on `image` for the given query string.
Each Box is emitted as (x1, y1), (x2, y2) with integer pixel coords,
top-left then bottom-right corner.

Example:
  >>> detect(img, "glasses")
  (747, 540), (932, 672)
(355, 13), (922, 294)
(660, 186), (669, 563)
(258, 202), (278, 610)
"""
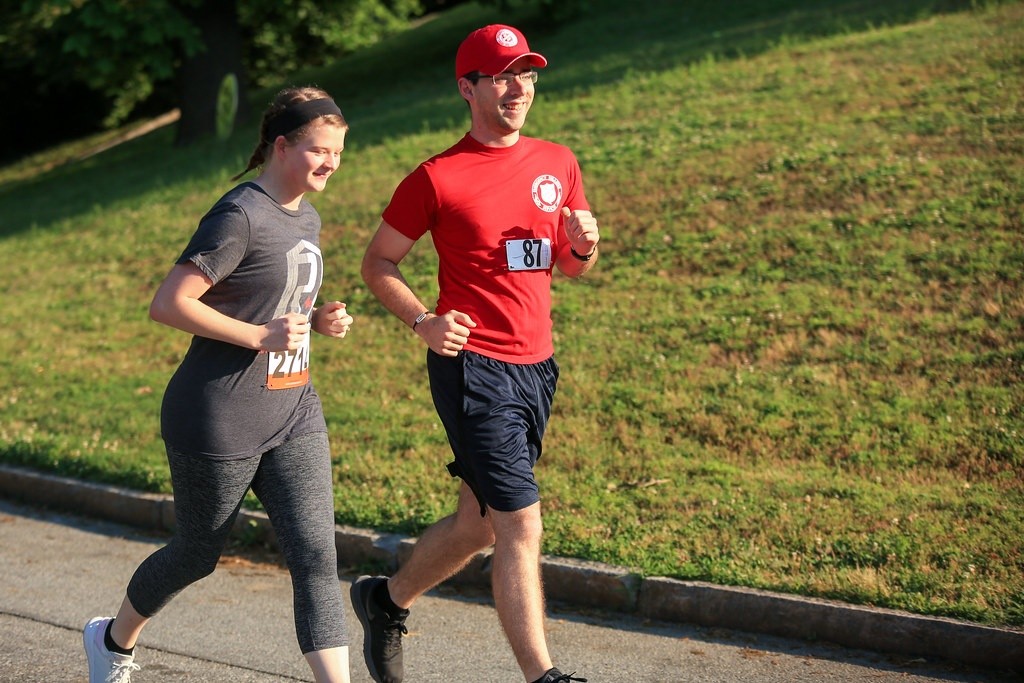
(479, 71), (539, 85)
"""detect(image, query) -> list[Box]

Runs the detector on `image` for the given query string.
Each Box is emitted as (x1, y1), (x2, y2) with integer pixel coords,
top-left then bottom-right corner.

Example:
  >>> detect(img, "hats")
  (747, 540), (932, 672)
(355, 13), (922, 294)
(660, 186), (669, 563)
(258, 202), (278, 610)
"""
(456, 24), (548, 81)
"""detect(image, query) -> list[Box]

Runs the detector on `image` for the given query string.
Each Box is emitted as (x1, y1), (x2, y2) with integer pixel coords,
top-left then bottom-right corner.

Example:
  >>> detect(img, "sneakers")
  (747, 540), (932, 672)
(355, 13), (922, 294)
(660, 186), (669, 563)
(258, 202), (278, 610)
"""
(533, 667), (587, 683)
(350, 575), (410, 683)
(82, 616), (141, 683)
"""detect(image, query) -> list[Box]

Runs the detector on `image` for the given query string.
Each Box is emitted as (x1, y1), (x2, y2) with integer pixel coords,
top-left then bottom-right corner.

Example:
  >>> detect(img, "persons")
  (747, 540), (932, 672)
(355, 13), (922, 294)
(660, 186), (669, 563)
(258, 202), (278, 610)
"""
(82, 86), (354, 682)
(347, 16), (599, 683)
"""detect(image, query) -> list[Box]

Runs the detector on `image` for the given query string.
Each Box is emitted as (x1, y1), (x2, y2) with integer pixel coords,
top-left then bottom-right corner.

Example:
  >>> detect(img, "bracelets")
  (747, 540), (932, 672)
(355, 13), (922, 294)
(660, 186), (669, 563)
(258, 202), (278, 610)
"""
(412, 310), (433, 333)
(570, 244), (596, 261)
